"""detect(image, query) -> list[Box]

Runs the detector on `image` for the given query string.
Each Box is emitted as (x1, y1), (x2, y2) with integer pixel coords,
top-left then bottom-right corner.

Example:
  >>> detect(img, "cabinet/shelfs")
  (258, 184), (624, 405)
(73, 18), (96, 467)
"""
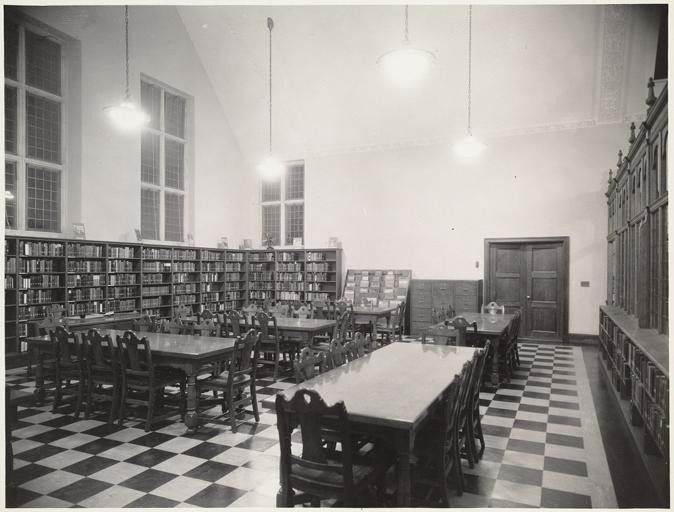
(596, 86), (668, 501)
(341, 269), (412, 336)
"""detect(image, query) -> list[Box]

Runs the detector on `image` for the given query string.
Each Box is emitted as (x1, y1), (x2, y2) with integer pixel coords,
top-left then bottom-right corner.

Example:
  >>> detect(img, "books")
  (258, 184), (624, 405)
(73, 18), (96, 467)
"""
(6, 238), (338, 352)
(343, 269), (409, 313)
